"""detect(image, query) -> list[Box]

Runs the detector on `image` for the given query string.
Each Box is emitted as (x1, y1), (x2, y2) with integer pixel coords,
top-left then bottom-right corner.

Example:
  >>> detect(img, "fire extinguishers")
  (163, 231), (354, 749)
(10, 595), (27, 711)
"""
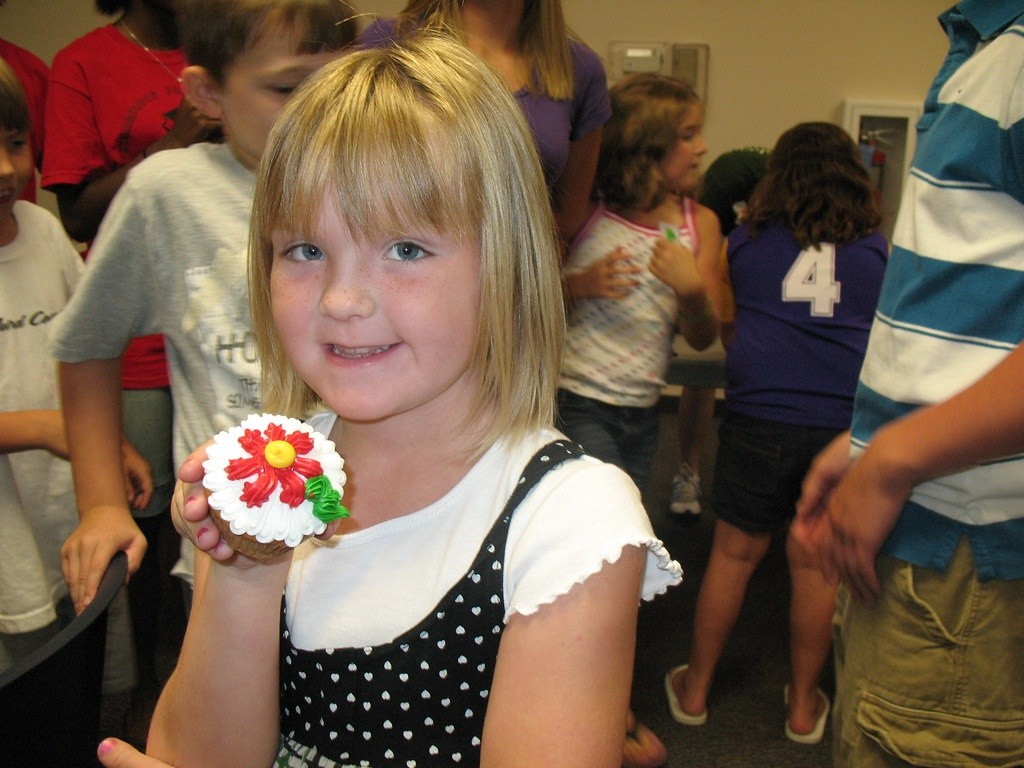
(859, 128), (897, 233)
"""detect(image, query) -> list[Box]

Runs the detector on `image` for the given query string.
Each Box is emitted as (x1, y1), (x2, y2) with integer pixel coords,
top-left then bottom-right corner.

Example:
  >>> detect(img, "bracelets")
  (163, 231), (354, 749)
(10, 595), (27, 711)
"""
(142, 148), (148, 157)
(678, 294), (712, 323)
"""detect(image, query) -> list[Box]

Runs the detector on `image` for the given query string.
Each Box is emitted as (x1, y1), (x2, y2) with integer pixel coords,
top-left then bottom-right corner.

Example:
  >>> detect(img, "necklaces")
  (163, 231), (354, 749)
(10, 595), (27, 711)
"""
(117, 17), (183, 84)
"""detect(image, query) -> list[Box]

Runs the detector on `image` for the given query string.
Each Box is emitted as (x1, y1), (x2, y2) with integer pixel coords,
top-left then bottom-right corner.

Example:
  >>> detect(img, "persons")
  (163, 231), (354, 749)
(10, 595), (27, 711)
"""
(556, 71), (729, 767)
(0, 1), (222, 767)
(354, 0), (611, 252)
(667, 121), (889, 744)
(788, 0), (1024, 768)
(54, 0), (361, 626)
(100, 31), (685, 768)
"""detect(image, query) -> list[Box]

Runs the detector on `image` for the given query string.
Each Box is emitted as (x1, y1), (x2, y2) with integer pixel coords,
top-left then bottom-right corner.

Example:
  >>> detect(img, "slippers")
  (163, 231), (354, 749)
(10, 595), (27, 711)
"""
(784, 683), (829, 745)
(665, 664), (707, 725)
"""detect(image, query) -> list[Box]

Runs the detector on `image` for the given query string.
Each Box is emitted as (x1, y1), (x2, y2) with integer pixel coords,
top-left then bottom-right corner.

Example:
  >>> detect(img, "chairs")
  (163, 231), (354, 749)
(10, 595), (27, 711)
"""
(0, 550), (128, 768)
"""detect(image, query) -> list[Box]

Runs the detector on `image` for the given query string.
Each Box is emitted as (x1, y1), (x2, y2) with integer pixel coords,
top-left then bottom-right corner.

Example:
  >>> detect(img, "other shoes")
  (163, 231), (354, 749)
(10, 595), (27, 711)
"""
(669, 477), (702, 520)
(671, 463), (701, 485)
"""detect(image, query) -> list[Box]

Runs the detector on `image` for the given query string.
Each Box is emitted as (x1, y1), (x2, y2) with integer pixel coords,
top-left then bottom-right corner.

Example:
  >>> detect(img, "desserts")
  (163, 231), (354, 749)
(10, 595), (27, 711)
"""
(202, 412), (350, 559)
